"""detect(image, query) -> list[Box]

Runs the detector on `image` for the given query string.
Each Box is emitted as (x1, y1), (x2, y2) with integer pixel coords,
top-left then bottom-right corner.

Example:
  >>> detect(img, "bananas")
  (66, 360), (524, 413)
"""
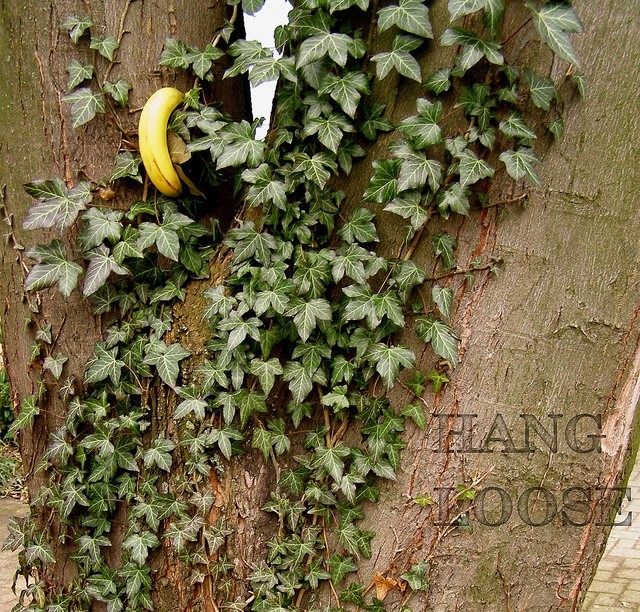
(138, 87), (185, 198)
